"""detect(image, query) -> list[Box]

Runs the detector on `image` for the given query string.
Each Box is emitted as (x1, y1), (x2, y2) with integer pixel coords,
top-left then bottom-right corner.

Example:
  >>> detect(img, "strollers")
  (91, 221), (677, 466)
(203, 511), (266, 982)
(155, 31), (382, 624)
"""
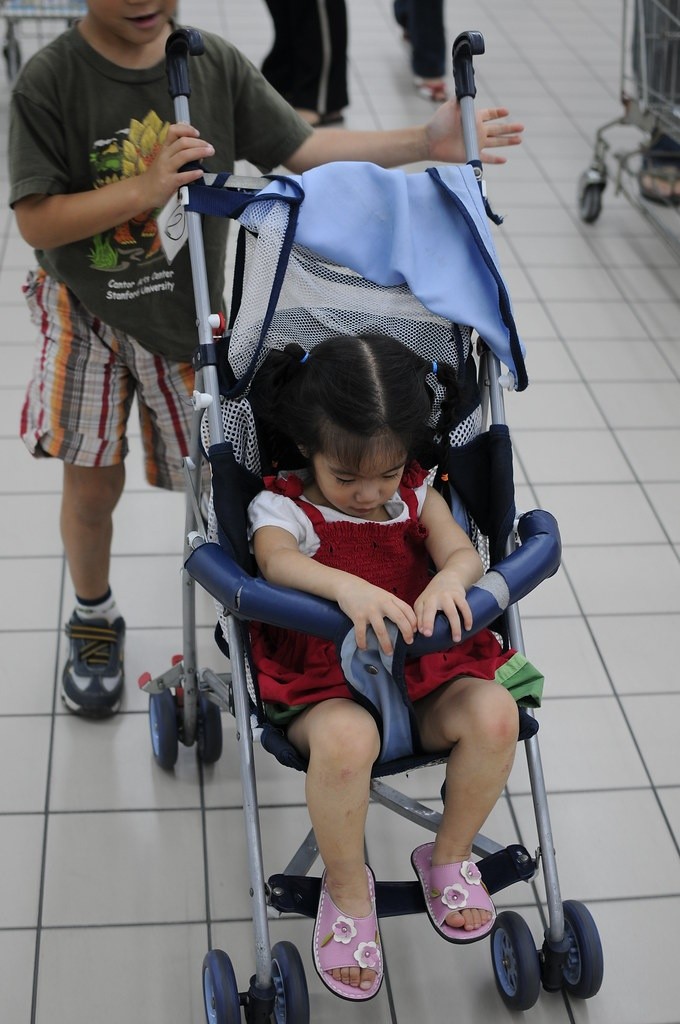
(138, 26), (604, 1024)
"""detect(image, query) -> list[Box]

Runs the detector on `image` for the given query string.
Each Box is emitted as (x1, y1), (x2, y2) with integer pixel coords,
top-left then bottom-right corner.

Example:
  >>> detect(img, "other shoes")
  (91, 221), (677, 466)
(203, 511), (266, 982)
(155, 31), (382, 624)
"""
(412, 75), (447, 102)
(310, 110), (343, 126)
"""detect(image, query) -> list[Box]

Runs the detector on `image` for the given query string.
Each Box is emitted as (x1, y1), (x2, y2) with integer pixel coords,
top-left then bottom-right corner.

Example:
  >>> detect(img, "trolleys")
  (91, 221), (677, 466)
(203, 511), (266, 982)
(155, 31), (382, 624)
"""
(1, 1), (92, 86)
(576, 1), (680, 225)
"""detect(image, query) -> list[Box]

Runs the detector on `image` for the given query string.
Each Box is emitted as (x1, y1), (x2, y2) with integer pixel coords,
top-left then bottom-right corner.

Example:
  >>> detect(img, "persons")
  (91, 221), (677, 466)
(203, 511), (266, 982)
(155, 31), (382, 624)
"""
(392, 0), (450, 103)
(6, 0), (524, 722)
(260, 0), (351, 127)
(246, 334), (520, 1001)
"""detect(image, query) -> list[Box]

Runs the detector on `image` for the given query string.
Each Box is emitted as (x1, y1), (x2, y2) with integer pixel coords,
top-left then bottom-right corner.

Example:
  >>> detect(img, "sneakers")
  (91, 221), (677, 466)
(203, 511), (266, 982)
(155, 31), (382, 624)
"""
(62, 609), (127, 720)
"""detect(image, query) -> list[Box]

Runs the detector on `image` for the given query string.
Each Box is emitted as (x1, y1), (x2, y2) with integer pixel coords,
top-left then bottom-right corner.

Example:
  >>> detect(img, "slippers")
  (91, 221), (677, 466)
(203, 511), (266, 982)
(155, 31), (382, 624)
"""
(312, 863), (384, 1002)
(411, 841), (497, 945)
